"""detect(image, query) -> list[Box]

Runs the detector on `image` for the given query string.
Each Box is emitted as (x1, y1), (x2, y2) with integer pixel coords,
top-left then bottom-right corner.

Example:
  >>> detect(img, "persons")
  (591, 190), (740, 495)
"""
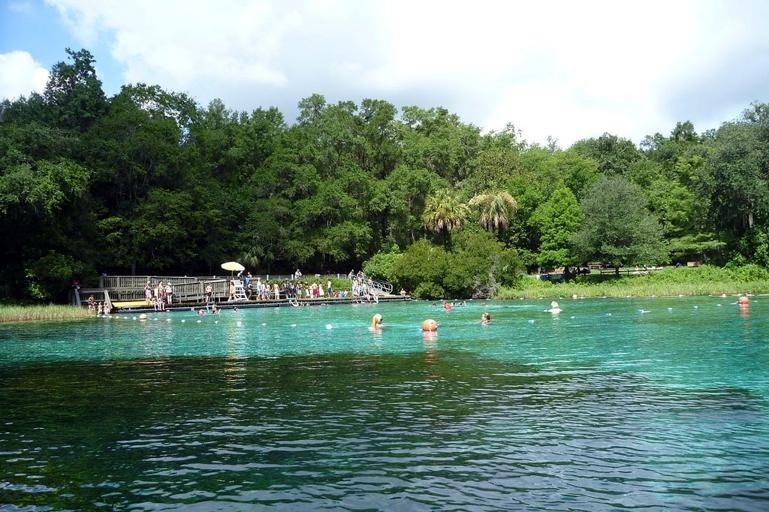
(205, 283), (218, 314)
(282, 270), (335, 299)
(400, 289), (407, 296)
(227, 272), (280, 301)
(88, 295), (111, 315)
(432, 297), (468, 312)
(372, 314), (384, 329)
(547, 301), (562, 313)
(335, 270), (374, 297)
(481, 313), (491, 325)
(143, 280), (173, 312)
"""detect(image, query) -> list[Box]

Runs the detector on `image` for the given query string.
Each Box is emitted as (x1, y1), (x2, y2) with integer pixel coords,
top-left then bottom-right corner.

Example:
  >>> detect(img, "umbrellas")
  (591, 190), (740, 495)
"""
(221, 261), (246, 276)
